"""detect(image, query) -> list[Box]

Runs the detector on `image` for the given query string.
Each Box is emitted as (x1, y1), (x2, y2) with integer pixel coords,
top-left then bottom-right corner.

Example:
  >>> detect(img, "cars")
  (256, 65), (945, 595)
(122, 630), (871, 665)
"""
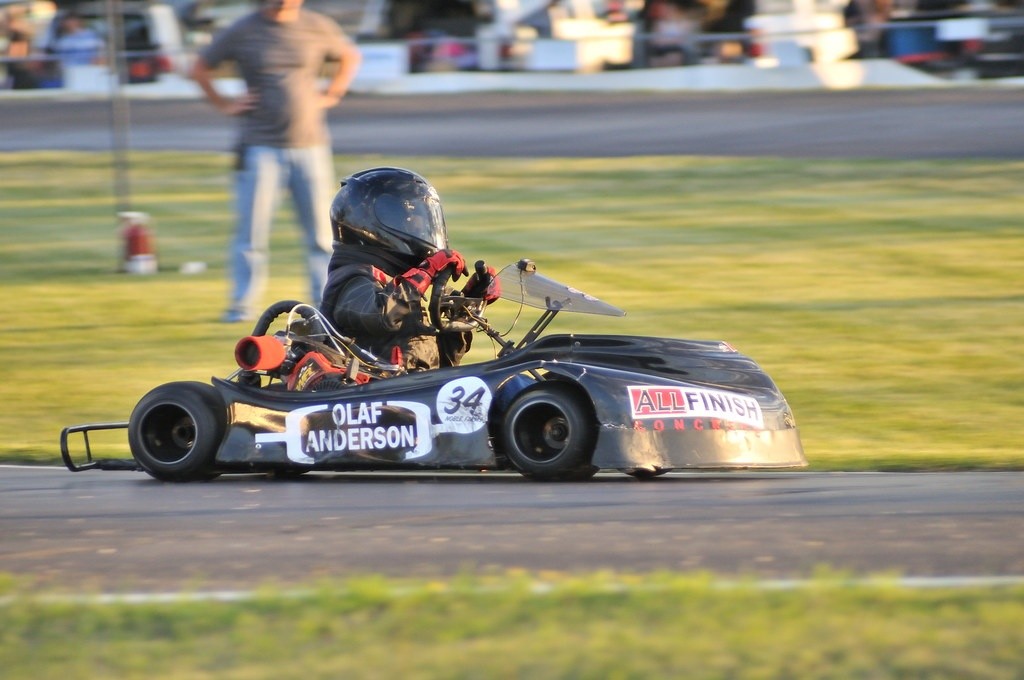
(32, 1), (1024, 89)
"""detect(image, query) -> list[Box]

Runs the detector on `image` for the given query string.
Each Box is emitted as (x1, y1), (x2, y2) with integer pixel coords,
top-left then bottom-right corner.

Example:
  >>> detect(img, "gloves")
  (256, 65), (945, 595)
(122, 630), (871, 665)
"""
(400, 249), (469, 295)
(461, 266), (501, 305)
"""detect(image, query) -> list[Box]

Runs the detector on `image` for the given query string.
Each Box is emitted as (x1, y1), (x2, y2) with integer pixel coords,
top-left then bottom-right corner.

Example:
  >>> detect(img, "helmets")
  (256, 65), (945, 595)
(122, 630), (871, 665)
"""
(330, 166), (449, 278)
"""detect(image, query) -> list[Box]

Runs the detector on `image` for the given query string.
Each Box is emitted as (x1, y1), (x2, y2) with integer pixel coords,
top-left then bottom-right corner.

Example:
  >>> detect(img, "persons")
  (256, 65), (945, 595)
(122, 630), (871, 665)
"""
(541, 0), (892, 68)
(198, 0), (364, 321)
(1, 0), (120, 91)
(320, 166), (500, 376)
